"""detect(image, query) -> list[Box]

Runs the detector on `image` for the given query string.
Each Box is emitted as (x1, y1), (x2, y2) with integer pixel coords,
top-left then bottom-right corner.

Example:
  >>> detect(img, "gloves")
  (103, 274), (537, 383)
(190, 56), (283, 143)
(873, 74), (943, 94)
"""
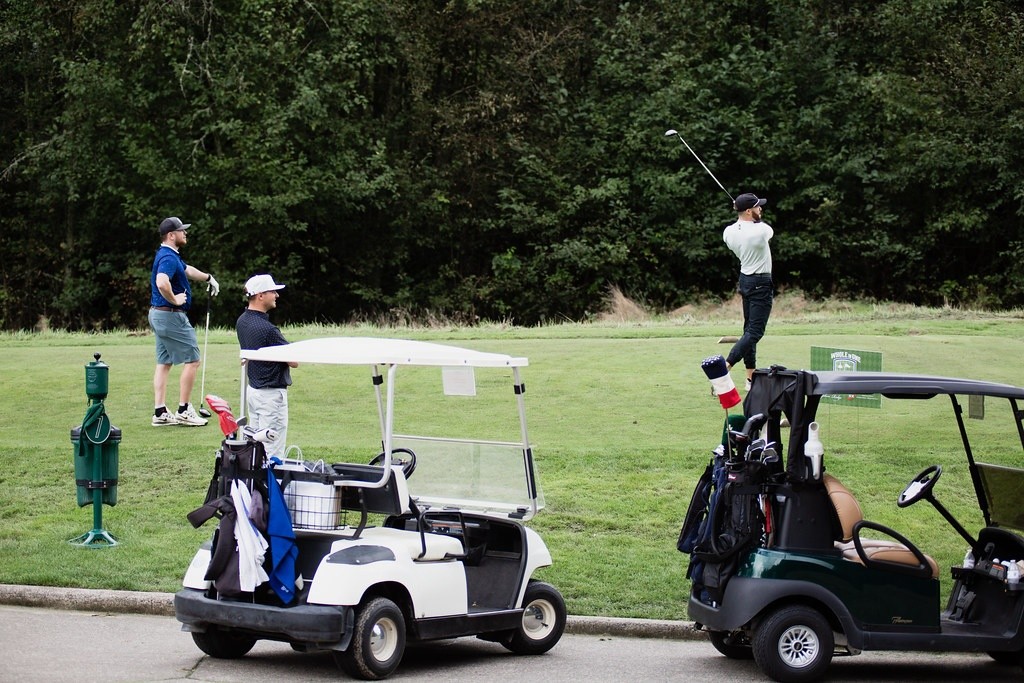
(206, 273), (220, 296)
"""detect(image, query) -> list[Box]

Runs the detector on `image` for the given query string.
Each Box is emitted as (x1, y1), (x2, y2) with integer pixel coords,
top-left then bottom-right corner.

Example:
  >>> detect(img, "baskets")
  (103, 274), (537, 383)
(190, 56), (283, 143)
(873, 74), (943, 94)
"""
(266, 469), (355, 530)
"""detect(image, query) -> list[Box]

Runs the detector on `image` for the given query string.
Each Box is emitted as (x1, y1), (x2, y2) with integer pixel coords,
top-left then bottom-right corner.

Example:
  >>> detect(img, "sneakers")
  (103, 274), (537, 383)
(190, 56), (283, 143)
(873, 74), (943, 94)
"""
(174, 403), (208, 426)
(152, 406), (179, 426)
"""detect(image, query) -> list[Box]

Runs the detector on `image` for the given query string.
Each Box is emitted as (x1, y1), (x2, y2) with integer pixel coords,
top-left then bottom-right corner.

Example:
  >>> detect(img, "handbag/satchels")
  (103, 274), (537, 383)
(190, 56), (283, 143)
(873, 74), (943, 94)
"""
(270, 444), (343, 530)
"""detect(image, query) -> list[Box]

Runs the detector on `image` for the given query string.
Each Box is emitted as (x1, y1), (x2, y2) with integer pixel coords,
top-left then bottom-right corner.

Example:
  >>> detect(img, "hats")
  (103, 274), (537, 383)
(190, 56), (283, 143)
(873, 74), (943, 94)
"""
(244, 274), (285, 296)
(735, 193), (767, 212)
(160, 216), (191, 235)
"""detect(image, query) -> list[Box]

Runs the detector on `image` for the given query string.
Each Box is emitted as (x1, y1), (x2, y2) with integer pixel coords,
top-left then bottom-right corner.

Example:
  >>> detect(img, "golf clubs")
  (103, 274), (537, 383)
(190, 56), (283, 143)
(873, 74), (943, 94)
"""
(665, 129), (734, 204)
(745, 438), (783, 464)
(235, 416), (265, 443)
(197, 288), (213, 419)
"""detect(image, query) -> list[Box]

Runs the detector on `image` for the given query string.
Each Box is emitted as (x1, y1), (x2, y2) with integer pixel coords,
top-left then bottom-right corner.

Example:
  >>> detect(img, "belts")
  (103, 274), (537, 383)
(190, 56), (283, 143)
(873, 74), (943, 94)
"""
(754, 273), (772, 277)
(152, 305), (184, 312)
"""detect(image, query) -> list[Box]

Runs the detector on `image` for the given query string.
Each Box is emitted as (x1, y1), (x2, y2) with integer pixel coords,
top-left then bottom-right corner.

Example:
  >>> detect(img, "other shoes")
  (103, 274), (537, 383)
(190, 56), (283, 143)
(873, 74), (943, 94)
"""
(745, 378), (752, 391)
(711, 386), (717, 397)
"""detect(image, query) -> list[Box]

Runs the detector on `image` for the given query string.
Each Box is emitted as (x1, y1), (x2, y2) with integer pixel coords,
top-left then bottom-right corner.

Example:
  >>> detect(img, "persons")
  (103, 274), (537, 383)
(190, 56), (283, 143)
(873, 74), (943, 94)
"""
(148, 218), (220, 426)
(236, 275), (297, 458)
(711, 193), (773, 394)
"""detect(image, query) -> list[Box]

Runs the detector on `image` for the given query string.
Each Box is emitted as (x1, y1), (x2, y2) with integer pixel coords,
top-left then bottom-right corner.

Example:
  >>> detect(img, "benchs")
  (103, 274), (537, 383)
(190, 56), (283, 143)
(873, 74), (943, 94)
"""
(269, 458), (470, 562)
(822, 472), (940, 580)
